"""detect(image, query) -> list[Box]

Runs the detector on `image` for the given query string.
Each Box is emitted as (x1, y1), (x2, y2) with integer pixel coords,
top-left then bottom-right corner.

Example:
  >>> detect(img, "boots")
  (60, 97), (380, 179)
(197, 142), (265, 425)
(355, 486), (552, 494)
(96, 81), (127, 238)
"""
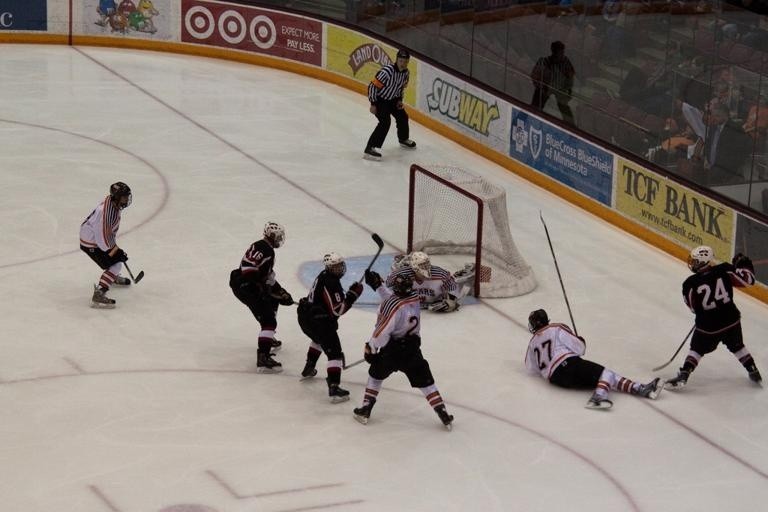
(664, 367), (690, 386)
(115, 275), (130, 286)
(638, 376), (660, 398)
(588, 391), (614, 407)
(353, 402), (376, 418)
(270, 337), (282, 347)
(747, 362), (762, 382)
(399, 138), (417, 148)
(434, 403), (454, 425)
(256, 349), (282, 368)
(363, 145), (382, 158)
(325, 377), (350, 397)
(90, 283), (117, 304)
(301, 361), (318, 378)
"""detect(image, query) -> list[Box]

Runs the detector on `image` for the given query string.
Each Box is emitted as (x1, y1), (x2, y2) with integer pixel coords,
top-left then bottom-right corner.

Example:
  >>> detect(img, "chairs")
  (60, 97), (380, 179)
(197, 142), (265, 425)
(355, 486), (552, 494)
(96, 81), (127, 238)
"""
(378, 1), (767, 197)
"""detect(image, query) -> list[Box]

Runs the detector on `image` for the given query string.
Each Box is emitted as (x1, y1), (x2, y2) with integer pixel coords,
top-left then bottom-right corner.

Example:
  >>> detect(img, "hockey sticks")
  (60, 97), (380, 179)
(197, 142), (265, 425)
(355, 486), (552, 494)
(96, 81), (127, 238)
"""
(652, 325), (695, 371)
(123, 261), (144, 283)
(359, 233), (383, 284)
(341, 352), (365, 370)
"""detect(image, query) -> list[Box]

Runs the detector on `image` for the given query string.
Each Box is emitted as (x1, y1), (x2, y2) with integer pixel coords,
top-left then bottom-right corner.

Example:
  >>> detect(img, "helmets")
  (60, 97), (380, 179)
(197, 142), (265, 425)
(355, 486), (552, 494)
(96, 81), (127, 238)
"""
(526, 309), (548, 333)
(323, 250), (347, 279)
(392, 274), (413, 298)
(687, 246), (713, 273)
(110, 182), (133, 210)
(263, 219), (285, 248)
(410, 251), (433, 278)
(396, 49), (412, 60)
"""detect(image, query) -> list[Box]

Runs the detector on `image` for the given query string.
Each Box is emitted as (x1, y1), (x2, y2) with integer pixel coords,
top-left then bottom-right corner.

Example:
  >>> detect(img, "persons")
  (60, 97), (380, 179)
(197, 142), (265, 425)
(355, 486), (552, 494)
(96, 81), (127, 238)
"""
(386, 251), (491, 313)
(229, 221), (294, 369)
(648, 97), (767, 186)
(353, 268), (453, 425)
(531, 40), (575, 124)
(297, 252), (363, 396)
(663, 246), (762, 388)
(524, 308), (661, 407)
(80, 182), (132, 304)
(364, 48), (416, 161)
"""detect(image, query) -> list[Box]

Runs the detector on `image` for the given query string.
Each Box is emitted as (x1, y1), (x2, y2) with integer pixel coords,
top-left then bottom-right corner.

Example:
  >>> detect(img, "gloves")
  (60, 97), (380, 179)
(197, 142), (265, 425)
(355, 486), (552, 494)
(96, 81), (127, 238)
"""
(274, 287), (294, 307)
(368, 269), (383, 291)
(732, 250), (744, 265)
(344, 281), (365, 302)
(363, 342), (375, 364)
(108, 243), (128, 264)
